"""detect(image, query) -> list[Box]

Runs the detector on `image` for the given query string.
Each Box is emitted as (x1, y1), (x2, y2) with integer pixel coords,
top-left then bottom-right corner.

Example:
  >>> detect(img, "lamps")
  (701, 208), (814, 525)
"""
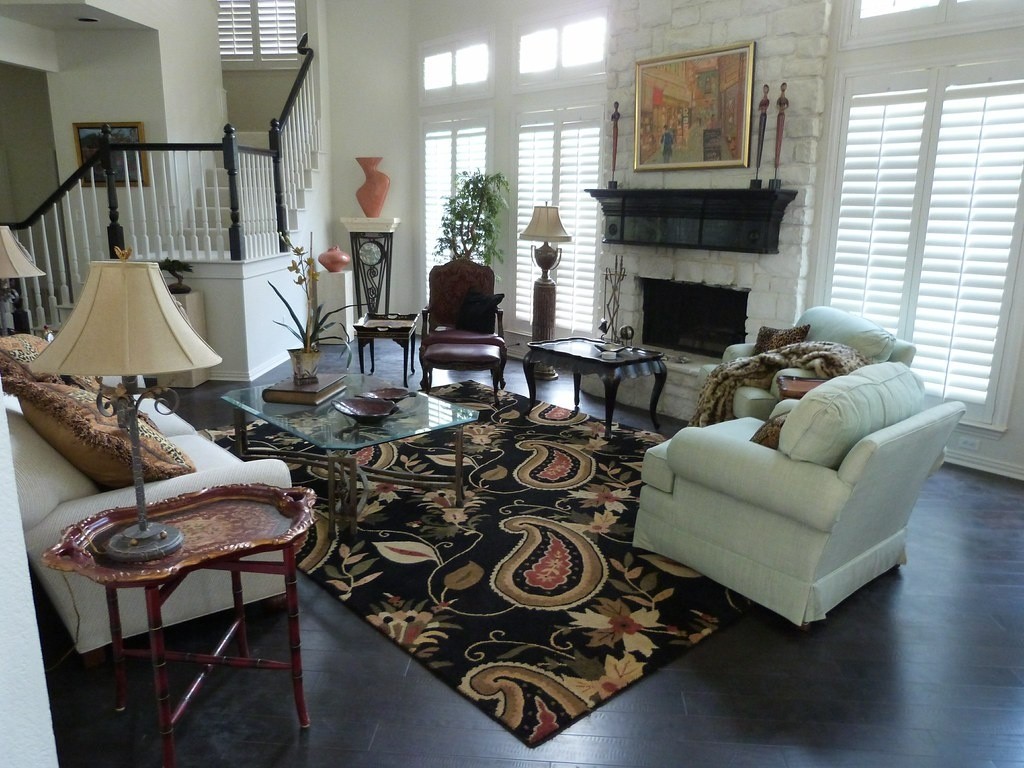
(519, 201), (572, 380)
(27, 245), (222, 563)
(0, 225), (47, 337)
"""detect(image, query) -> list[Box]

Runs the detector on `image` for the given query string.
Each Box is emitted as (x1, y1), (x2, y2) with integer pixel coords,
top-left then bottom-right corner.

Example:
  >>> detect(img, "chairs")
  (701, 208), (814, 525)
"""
(417, 257), (508, 406)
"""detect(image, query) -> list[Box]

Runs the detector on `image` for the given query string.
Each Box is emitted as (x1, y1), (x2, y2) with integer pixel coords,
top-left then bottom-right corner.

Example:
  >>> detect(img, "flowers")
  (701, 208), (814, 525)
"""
(270, 229), (375, 372)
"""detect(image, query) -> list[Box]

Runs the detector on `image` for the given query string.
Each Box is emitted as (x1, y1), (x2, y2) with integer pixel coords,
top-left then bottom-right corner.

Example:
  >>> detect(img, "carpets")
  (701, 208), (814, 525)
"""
(195, 379), (759, 748)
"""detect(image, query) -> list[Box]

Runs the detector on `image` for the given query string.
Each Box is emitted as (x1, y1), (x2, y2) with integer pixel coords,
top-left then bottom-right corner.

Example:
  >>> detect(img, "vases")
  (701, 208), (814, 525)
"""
(317, 246), (352, 271)
(355, 157), (390, 218)
(287, 349), (321, 387)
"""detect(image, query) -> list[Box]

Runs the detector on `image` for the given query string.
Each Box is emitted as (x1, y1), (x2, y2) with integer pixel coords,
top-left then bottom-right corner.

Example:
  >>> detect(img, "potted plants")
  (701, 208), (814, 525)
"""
(157, 256), (195, 293)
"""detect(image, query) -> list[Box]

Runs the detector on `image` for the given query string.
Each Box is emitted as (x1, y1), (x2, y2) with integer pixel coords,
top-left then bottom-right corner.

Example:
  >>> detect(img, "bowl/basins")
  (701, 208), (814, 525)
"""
(332, 398), (399, 424)
(354, 388), (417, 404)
(594, 343), (625, 354)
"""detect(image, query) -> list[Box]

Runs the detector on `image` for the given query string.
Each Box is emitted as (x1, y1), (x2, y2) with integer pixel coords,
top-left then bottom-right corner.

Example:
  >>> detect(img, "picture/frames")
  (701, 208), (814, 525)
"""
(71, 122), (150, 187)
(633, 39), (756, 172)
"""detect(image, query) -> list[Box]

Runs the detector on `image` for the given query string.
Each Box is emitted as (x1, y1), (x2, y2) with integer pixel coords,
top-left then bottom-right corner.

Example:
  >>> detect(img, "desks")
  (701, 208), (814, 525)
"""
(522, 337), (669, 440)
(355, 310), (420, 389)
(42, 482), (317, 768)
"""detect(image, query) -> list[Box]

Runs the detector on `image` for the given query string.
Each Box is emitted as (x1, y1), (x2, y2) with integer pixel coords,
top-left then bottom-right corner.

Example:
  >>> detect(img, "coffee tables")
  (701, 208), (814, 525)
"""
(221, 372), (480, 541)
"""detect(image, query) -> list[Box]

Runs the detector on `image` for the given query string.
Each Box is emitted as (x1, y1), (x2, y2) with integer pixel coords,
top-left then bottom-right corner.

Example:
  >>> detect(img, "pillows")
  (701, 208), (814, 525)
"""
(753, 324), (812, 356)
(17, 382), (196, 488)
(751, 410), (790, 450)
(0, 333), (110, 399)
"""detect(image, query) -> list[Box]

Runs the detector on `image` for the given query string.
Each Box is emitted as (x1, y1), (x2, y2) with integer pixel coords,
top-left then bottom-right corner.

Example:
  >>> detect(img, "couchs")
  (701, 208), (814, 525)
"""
(631, 362), (967, 634)
(697, 303), (915, 422)
